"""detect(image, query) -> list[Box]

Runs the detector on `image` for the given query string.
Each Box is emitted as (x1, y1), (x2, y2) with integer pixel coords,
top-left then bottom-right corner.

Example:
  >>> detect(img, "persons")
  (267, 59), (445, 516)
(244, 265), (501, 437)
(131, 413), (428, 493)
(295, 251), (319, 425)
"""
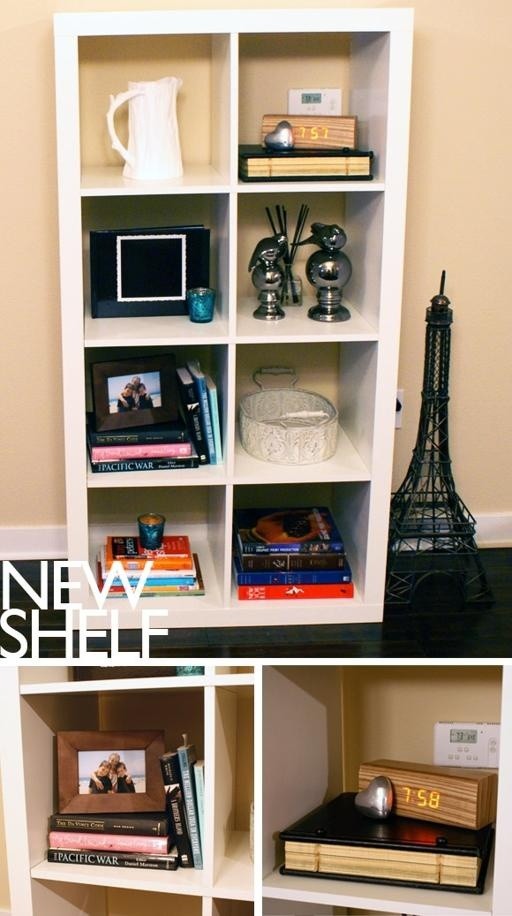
(231, 506), (354, 600)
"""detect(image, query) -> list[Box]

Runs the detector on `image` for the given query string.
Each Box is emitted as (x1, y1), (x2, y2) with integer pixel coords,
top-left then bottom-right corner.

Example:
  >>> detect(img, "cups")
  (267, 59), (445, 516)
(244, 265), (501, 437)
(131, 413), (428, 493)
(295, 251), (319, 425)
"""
(136, 513), (165, 549)
(277, 276), (303, 306)
(188, 289), (214, 324)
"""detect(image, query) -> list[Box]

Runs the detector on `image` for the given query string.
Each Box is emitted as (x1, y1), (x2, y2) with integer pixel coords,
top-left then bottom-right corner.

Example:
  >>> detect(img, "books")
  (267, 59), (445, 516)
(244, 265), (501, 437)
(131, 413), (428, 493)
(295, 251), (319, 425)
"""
(239, 144), (374, 181)
(48, 744), (205, 871)
(87, 359), (222, 473)
(95, 535), (205, 598)
(279, 792), (495, 894)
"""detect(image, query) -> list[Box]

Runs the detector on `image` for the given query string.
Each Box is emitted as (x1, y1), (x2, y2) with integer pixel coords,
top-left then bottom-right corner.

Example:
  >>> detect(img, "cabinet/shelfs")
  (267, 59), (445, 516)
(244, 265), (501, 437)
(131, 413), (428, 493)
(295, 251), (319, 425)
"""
(1, 654), (508, 916)
(56, 7), (418, 635)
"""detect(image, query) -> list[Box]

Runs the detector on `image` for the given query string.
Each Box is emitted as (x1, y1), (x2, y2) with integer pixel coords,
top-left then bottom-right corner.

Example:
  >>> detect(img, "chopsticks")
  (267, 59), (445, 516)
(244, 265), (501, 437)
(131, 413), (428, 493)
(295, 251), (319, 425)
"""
(263, 203), (309, 303)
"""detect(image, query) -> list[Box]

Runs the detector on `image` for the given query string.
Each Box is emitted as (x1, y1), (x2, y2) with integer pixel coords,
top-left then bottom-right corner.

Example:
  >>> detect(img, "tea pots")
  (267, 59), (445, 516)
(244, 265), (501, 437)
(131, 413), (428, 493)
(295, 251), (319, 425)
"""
(107, 77), (189, 184)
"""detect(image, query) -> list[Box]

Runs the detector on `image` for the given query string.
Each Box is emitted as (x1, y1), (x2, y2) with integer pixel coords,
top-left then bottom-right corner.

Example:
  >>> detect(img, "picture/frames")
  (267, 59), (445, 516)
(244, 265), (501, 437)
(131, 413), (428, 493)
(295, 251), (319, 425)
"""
(91, 354), (181, 430)
(55, 731), (168, 815)
